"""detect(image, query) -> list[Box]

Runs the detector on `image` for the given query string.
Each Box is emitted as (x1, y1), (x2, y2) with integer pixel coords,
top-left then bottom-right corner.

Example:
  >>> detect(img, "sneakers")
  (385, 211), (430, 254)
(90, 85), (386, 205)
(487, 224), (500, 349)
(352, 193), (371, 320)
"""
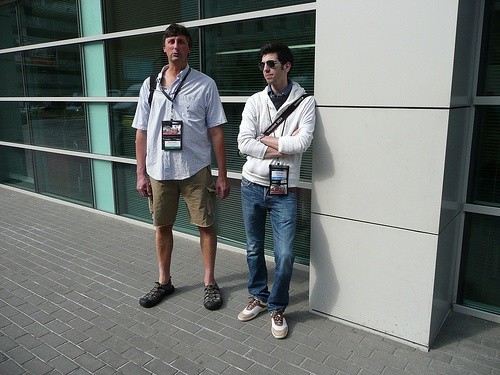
(204, 282), (223, 311)
(237, 296), (269, 322)
(269, 311), (289, 338)
(139, 275), (175, 308)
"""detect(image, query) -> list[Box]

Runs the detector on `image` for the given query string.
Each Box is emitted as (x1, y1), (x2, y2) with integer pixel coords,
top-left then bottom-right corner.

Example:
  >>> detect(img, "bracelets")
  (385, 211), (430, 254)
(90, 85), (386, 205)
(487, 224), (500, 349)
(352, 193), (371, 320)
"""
(256, 133), (265, 142)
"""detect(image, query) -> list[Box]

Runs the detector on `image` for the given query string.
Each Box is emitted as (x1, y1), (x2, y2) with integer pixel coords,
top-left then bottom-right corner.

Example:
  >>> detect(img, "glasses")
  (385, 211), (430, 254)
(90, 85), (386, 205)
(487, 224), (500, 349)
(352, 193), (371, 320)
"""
(258, 60), (287, 71)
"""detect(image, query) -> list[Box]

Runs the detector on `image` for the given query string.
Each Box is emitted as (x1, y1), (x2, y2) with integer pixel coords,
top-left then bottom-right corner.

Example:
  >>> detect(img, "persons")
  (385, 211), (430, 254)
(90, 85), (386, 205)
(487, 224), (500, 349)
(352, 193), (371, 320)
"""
(132, 21), (230, 312)
(237, 36), (318, 339)
